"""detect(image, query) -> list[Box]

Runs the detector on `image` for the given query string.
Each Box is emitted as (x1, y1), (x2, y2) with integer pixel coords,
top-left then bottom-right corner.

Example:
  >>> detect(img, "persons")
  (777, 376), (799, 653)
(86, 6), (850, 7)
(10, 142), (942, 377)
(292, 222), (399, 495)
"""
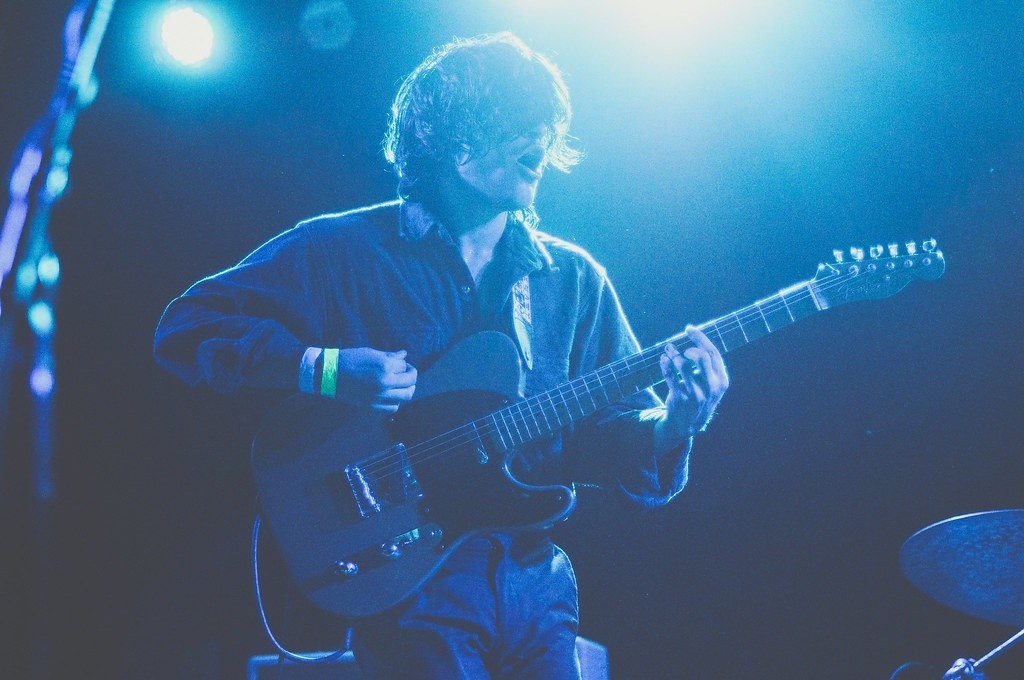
(152, 32), (729, 680)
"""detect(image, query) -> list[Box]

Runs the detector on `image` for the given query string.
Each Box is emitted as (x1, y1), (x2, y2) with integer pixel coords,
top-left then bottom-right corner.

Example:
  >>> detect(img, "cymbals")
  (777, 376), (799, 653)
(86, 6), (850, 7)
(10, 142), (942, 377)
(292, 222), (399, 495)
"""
(899, 505), (1024, 626)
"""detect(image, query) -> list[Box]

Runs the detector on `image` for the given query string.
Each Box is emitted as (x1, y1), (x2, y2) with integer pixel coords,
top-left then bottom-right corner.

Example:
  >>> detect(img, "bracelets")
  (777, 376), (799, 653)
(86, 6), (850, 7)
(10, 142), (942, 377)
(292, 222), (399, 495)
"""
(320, 348), (339, 398)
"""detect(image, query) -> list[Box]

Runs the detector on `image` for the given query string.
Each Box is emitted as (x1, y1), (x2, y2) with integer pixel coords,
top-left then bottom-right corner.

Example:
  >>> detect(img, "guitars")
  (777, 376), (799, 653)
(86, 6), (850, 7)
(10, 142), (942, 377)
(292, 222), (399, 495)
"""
(252, 236), (947, 618)
